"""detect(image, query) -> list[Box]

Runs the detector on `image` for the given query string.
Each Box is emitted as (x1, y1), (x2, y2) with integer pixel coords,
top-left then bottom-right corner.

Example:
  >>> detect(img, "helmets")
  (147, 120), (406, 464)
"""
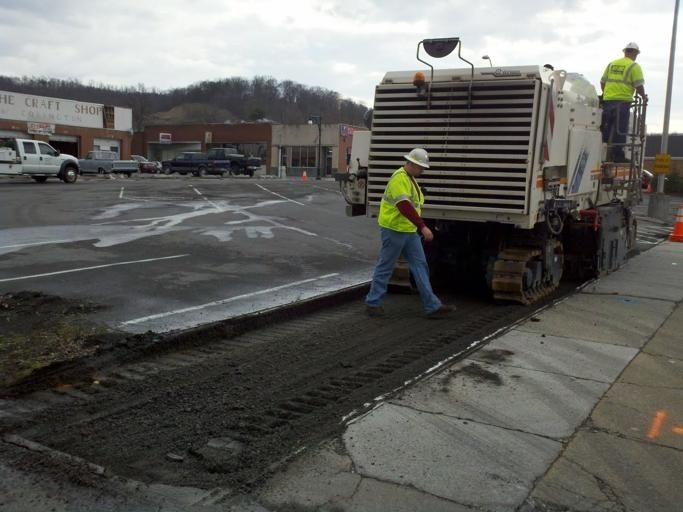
(623, 42), (640, 54)
(403, 147), (430, 169)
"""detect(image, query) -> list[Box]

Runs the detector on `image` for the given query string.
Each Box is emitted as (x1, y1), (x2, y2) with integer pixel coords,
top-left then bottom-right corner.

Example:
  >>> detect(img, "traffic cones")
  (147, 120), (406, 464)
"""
(301, 170), (309, 182)
(668, 205), (683, 243)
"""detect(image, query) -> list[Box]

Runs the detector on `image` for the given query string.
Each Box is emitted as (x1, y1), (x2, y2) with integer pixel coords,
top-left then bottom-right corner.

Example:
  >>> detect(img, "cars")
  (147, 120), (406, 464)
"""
(642, 169), (668, 188)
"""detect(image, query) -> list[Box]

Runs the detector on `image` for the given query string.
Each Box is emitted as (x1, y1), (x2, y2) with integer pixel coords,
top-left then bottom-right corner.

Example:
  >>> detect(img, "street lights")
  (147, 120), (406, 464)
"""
(482, 54), (492, 67)
(308, 116), (322, 179)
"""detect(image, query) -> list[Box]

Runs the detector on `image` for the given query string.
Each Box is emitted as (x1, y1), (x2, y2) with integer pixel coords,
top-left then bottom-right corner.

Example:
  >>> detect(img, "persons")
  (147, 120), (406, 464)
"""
(597, 41), (647, 163)
(361, 147), (458, 320)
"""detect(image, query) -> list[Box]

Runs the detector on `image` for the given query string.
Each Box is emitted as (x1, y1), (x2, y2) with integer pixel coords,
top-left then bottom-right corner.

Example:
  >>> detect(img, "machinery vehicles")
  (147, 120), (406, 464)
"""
(333, 37), (648, 307)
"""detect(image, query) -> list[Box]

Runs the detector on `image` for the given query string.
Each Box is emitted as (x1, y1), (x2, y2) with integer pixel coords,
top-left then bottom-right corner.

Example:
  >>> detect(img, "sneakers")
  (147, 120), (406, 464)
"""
(426, 305), (456, 316)
(366, 305), (384, 314)
(613, 158), (631, 163)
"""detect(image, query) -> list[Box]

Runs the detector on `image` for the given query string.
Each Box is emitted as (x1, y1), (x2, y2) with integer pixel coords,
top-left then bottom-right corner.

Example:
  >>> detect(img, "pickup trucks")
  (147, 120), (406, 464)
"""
(0, 139), (262, 184)
